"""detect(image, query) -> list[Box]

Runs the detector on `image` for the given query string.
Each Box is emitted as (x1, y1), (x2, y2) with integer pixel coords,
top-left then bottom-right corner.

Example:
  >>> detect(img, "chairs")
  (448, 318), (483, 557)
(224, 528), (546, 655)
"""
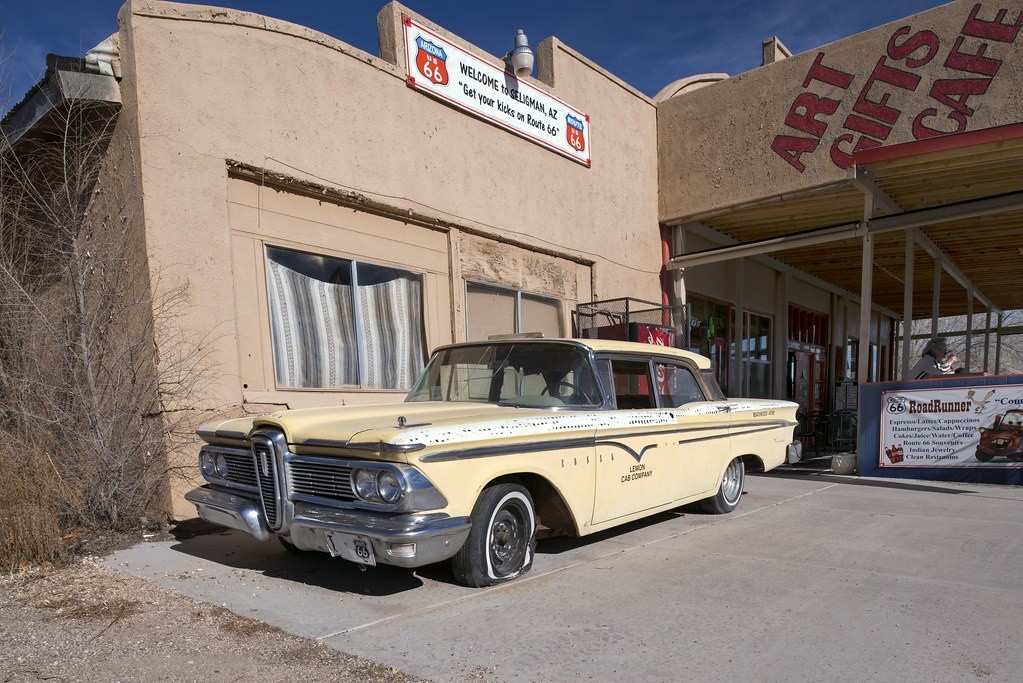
(796, 409), (857, 457)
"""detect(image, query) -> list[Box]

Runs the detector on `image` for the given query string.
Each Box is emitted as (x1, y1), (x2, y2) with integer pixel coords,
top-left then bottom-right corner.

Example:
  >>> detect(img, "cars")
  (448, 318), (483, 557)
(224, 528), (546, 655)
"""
(184, 331), (802, 588)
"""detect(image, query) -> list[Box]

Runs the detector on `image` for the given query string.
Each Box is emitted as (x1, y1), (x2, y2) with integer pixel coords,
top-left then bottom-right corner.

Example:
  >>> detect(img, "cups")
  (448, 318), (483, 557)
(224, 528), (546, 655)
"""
(945, 350), (957, 362)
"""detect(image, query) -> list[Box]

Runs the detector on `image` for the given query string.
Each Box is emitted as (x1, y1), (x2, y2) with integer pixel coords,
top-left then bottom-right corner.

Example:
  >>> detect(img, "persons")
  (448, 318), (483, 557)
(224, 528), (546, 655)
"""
(908, 337), (957, 380)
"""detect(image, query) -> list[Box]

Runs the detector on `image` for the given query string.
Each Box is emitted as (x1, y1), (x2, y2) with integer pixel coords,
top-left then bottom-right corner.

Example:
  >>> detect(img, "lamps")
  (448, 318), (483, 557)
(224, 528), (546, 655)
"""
(500, 29), (535, 78)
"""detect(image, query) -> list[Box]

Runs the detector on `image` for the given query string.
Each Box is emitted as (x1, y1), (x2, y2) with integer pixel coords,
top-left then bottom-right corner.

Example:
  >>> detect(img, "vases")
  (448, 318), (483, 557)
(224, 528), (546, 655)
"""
(831, 454), (855, 475)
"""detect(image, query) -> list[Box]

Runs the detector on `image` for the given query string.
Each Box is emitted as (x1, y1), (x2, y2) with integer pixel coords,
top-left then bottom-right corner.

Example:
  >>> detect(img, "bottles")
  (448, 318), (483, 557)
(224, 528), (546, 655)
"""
(885, 447), (894, 464)
(897, 444), (903, 461)
(892, 445), (896, 463)
